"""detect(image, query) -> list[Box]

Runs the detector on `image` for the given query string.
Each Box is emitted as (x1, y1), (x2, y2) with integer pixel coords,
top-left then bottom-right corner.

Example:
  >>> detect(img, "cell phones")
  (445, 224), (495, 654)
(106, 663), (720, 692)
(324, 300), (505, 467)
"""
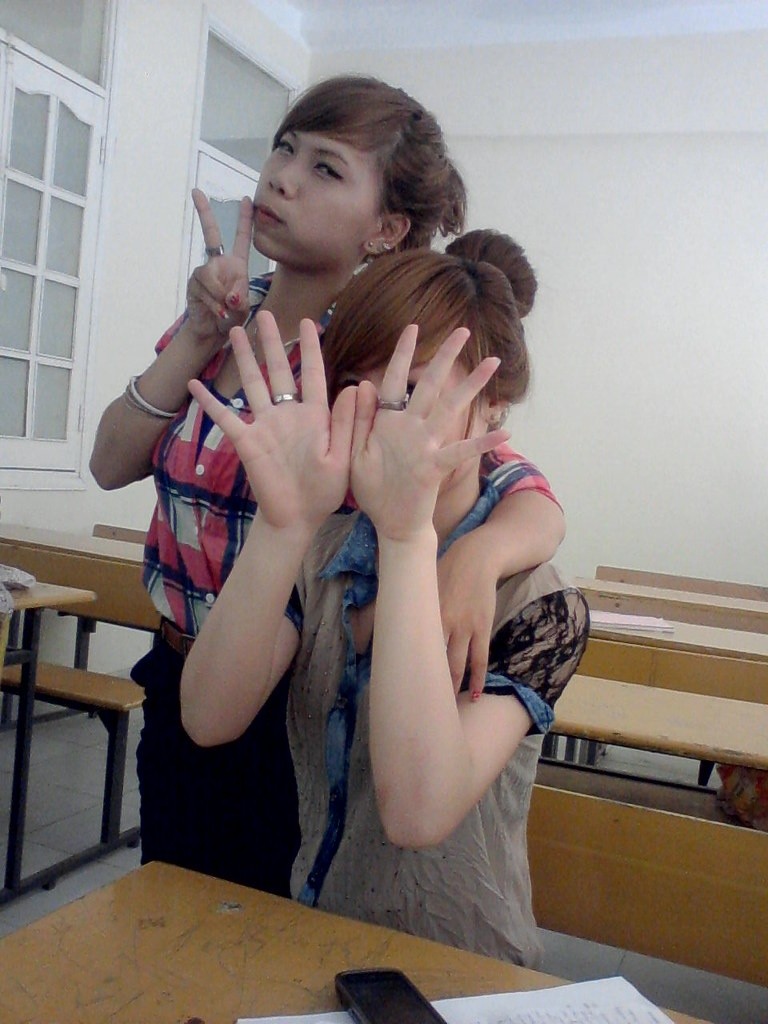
(334, 967), (448, 1024)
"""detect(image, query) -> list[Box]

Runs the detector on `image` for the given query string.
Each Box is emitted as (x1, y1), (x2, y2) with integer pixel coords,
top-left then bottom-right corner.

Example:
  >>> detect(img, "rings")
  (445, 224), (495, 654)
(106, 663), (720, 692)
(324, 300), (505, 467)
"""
(380, 401), (402, 410)
(273, 393), (297, 404)
(205, 244), (224, 256)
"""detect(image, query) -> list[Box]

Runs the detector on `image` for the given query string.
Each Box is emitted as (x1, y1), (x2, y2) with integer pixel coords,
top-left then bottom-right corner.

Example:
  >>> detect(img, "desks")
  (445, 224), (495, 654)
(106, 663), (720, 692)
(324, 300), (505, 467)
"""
(0, 525), (768, 1024)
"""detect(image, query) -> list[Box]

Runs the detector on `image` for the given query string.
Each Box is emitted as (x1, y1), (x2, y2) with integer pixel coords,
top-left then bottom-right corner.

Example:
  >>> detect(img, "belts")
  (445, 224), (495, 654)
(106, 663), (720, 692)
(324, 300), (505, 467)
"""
(160, 618), (198, 664)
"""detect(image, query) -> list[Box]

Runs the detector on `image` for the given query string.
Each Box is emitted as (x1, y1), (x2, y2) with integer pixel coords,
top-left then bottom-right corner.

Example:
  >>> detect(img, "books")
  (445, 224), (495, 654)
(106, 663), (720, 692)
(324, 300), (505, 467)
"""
(589, 611), (675, 633)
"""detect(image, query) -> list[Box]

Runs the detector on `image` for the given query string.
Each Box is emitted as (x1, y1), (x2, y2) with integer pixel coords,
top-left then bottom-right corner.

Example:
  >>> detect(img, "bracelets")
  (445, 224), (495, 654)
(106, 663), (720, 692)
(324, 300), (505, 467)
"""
(123, 376), (177, 420)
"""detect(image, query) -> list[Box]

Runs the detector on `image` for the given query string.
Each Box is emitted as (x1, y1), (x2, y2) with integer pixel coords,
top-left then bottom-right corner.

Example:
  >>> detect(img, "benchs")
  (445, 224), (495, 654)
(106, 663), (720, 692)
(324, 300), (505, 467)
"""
(0, 658), (147, 887)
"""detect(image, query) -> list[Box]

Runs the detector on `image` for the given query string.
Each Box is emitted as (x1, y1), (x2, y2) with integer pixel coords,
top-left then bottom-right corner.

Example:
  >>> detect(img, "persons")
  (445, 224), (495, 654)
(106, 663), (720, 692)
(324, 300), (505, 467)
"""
(88, 80), (566, 900)
(180, 228), (590, 971)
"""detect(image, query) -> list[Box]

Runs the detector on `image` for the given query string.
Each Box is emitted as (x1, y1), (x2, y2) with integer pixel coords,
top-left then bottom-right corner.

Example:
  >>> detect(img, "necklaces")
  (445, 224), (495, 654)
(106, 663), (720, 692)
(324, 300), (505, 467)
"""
(251, 328), (300, 358)
